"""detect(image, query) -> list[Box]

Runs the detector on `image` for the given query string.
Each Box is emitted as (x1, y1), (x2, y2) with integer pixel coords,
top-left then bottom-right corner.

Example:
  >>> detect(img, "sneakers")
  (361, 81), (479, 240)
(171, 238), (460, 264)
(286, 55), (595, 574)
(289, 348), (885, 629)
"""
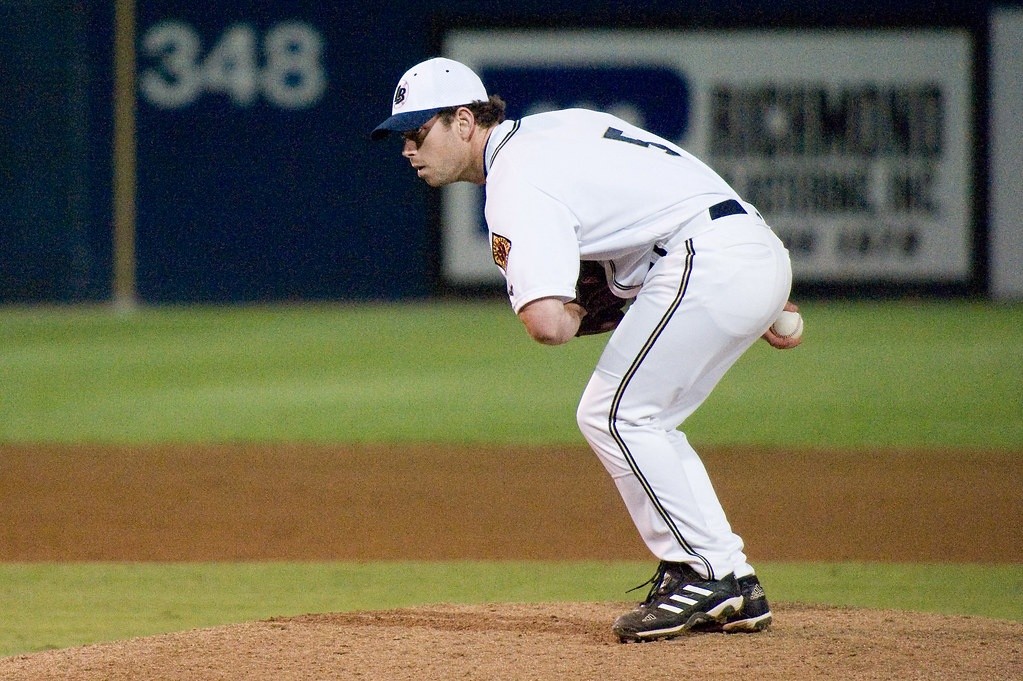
(612, 560), (773, 643)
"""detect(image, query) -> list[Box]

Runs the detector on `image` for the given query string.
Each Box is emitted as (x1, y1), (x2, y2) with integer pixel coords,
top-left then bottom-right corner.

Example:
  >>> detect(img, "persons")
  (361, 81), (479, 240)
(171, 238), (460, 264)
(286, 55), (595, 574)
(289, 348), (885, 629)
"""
(370, 58), (803, 643)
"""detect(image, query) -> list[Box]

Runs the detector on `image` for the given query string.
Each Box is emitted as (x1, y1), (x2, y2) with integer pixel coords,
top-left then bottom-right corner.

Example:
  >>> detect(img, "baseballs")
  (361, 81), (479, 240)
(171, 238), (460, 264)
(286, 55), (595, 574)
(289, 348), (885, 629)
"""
(770, 311), (804, 339)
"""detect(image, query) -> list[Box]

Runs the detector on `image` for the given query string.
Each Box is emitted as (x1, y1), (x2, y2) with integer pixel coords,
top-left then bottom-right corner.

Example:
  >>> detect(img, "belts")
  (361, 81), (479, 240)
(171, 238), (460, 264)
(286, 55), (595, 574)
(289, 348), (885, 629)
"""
(649, 200), (747, 270)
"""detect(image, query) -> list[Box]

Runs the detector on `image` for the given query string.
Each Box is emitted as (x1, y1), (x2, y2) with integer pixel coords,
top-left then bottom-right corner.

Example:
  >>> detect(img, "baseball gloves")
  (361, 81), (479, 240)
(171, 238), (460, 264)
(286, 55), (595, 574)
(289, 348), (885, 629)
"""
(564, 259), (627, 337)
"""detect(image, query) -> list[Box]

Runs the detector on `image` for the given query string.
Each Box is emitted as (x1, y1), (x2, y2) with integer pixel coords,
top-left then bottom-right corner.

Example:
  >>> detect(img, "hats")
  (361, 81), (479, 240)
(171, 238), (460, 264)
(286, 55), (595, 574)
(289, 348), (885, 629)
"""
(370, 56), (491, 134)
